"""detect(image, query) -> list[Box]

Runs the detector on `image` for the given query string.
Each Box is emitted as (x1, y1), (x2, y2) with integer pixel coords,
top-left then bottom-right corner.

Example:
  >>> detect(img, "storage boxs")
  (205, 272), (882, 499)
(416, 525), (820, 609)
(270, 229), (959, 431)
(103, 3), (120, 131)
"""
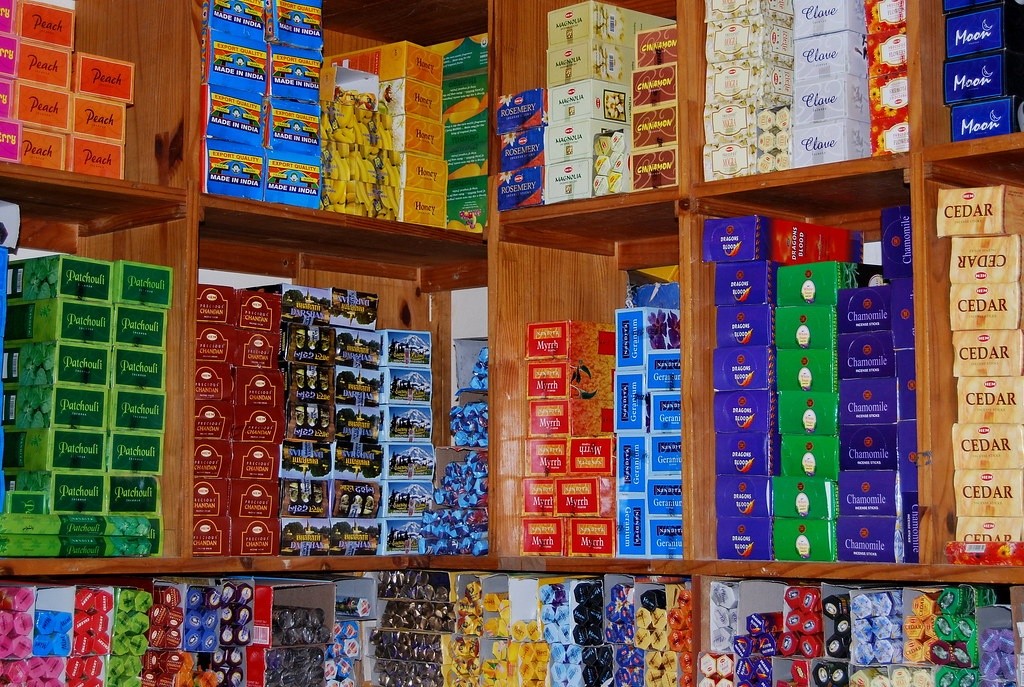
(0, 0), (1024, 685)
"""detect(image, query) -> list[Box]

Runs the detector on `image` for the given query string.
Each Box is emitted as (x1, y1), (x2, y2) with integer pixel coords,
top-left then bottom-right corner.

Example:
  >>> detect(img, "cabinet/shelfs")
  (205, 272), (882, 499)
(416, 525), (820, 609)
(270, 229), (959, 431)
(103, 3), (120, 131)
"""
(491, 1), (1023, 685)
(0, 0), (491, 686)
